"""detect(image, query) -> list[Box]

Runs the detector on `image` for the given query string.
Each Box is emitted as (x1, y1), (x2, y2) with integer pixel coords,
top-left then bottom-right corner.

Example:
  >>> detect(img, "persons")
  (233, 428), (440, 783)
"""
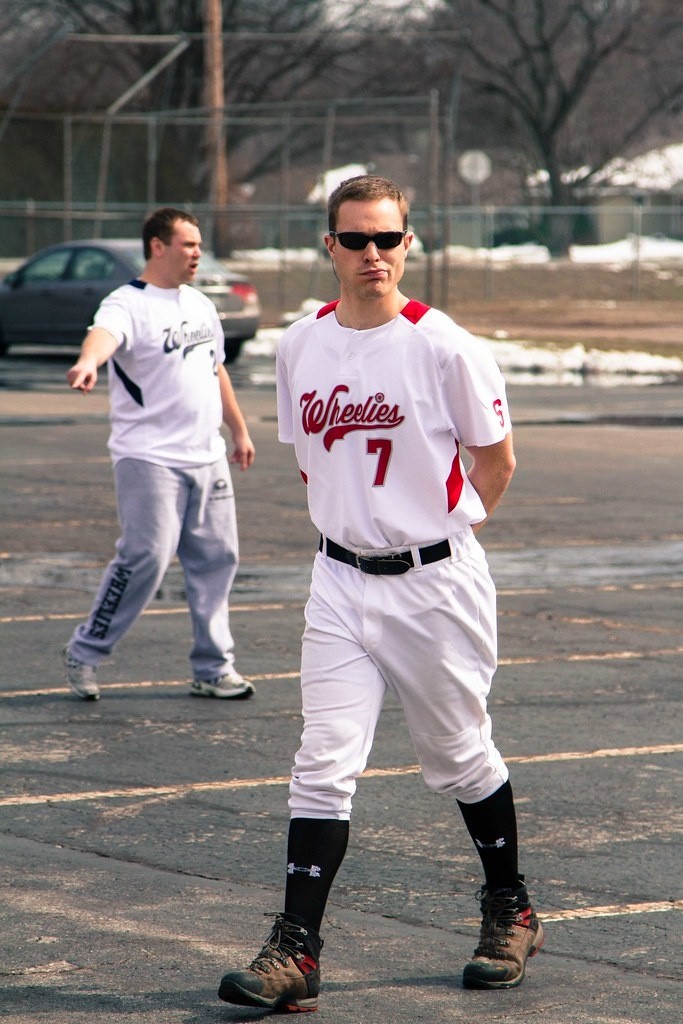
(214, 173), (543, 1009)
(56, 209), (257, 701)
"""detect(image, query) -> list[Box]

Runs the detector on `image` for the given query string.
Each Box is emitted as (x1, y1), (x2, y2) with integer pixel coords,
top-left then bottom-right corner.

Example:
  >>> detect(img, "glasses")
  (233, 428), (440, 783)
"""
(330, 230), (407, 250)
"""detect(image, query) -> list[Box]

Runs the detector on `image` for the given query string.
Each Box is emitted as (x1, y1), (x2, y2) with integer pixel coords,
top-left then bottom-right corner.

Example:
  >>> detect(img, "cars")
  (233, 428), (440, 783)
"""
(1, 237), (263, 368)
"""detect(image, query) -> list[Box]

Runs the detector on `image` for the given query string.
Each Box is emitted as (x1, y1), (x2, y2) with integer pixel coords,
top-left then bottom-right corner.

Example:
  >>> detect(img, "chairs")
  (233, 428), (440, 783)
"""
(83, 264), (108, 279)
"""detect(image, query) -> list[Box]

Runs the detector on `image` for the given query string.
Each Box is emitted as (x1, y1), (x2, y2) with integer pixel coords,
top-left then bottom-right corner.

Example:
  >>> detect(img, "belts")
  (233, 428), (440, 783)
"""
(319, 532), (454, 575)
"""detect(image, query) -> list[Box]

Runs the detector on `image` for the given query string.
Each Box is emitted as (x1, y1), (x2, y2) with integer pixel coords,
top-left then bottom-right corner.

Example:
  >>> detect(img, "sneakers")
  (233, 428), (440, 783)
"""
(217, 910), (324, 1012)
(462, 876), (544, 989)
(62, 644), (101, 700)
(190, 670), (255, 699)
